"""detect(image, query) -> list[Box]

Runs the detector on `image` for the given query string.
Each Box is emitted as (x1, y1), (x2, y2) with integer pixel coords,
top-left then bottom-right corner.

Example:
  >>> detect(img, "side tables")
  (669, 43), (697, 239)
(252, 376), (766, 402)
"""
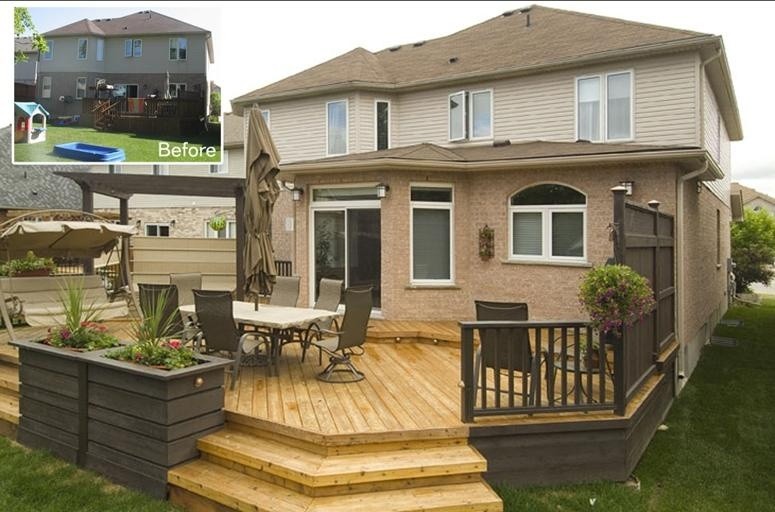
(552, 360), (607, 407)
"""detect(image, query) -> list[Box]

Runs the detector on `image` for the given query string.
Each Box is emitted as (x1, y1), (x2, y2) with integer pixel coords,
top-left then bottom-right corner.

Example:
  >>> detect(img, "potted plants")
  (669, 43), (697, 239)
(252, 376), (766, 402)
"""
(6, 273), (236, 501)
(579, 336), (608, 370)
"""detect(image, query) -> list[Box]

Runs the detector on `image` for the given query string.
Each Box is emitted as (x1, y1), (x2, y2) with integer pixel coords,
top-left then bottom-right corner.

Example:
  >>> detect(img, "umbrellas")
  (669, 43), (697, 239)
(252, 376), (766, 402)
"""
(243, 99), (281, 359)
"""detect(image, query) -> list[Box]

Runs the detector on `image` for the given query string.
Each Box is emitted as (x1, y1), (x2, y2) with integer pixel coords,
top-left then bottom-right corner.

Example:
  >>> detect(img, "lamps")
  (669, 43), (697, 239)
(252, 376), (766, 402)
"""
(618, 180), (634, 196)
(291, 187), (304, 202)
(375, 182), (389, 199)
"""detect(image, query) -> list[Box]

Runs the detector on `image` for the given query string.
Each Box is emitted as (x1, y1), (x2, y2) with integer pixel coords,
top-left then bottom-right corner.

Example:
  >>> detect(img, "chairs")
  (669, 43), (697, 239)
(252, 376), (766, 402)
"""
(470, 298), (551, 417)
(137, 272), (376, 384)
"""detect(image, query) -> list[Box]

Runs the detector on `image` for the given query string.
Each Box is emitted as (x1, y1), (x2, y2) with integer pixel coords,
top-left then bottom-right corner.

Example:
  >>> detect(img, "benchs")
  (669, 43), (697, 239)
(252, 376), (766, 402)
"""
(0, 244), (129, 327)
